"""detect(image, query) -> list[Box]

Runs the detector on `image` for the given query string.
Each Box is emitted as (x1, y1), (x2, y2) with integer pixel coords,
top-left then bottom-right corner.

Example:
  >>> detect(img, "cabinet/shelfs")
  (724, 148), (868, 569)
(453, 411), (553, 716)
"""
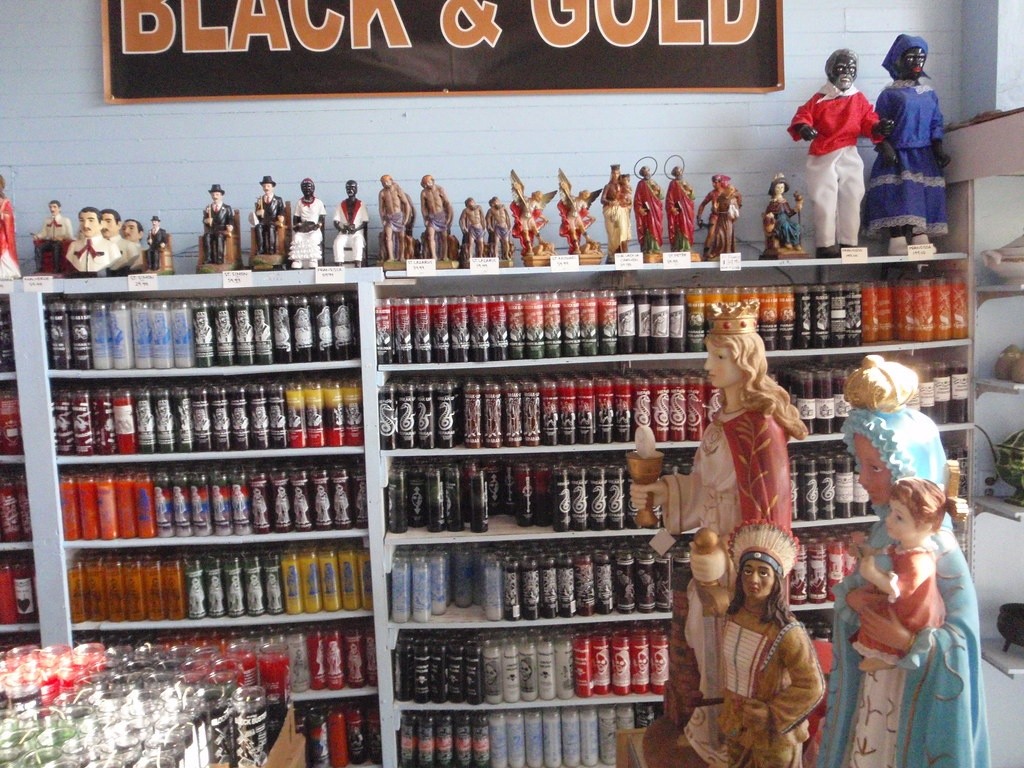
(1, 268), (401, 768)
(373, 251), (977, 768)
(930, 104), (1024, 679)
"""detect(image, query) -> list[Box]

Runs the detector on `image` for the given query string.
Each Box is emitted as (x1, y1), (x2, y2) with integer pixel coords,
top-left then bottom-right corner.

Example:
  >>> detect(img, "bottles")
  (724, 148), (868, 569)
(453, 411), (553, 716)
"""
(1, 274), (969, 768)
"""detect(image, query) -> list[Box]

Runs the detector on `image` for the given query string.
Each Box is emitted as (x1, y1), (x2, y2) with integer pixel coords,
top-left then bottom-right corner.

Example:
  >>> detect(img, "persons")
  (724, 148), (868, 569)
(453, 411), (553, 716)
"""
(676, 527), (827, 767)
(1, 35), (953, 277)
(854, 482), (963, 673)
(632, 327), (806, 767)
(818, 406), (989, 768)
